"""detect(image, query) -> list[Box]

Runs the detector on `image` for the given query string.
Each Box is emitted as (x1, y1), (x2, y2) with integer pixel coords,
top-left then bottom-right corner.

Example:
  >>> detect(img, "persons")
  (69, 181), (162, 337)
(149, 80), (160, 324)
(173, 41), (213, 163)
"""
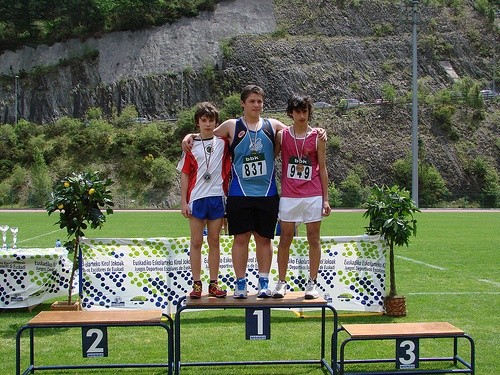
(181, 85), (327, 299)
(176, 102), (231, 299)
(272, 95), (331, 298)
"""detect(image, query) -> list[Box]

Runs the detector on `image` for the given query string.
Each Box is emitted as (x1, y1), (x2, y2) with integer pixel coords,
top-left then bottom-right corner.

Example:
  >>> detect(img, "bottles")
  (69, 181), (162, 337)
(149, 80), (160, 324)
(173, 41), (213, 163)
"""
(55, 239), (61, 253)
(203, 228), (207, 237)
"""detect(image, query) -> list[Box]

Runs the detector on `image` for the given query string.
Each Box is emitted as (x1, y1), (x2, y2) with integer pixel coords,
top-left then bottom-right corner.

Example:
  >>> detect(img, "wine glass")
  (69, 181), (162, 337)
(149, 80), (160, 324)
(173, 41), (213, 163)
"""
(0, 225), (9, 250)
(10, 226), (19, 248)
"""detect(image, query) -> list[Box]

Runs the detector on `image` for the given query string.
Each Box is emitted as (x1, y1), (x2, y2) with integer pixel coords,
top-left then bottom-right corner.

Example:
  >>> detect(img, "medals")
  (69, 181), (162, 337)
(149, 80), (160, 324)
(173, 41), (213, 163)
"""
(250, 150), (258, 158)
(296, 164), (304, 172)
(203, 173), (211, 182)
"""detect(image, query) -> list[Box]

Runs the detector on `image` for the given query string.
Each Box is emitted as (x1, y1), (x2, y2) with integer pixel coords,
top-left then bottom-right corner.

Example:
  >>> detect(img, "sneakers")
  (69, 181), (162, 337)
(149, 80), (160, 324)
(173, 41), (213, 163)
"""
(234, 279), (248, 299)
(257, 277), (271, 298)
(190, 283), (203, 299)
(208, 281), (227, 297)
(305, 280), (319, 299)
(271, 280), (287, 297)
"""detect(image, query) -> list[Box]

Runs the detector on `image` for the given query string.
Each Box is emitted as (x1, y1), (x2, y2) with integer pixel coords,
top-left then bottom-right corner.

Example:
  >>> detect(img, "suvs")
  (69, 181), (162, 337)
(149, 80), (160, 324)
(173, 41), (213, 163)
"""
(340, 98), (365, 109)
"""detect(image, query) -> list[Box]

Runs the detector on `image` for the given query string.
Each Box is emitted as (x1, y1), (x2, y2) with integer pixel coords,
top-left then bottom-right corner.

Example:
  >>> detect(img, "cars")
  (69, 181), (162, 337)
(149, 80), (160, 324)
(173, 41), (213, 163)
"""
(480, 90), (500, 100)
(375, 98), (390, 105)
(312, 101), (335, 110)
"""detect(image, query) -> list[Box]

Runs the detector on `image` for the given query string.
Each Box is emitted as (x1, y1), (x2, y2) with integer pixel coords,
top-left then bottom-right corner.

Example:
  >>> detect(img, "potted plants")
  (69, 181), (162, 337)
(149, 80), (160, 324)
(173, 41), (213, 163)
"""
(365, 185), (421, 316)
(44, 169), (113, 311)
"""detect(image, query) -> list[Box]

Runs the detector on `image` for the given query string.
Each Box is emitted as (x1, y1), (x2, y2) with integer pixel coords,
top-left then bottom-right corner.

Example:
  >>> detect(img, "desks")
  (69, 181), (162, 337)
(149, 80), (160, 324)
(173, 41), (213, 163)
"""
(0, 248), (80, 312)
